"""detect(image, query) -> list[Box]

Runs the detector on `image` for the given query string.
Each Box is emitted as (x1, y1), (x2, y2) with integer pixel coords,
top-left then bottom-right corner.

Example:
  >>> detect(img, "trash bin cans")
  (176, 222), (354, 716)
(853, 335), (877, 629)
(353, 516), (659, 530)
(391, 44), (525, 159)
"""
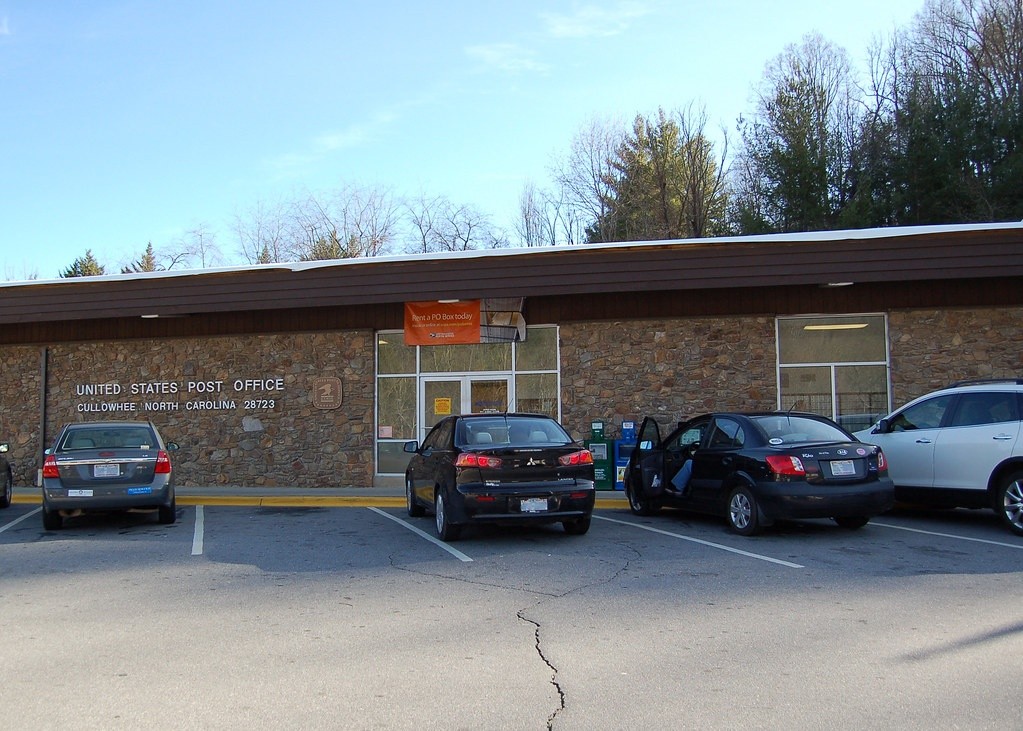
(614, 421), (640, 491)
(584, 420), (614, 490)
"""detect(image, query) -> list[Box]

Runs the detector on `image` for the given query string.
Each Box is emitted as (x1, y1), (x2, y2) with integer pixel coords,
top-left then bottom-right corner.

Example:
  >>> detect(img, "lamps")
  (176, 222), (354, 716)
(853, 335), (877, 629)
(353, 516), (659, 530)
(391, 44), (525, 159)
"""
(141, 315), (191, 318)
(438, 299), (474, 304)
(817, 283), (854, 287)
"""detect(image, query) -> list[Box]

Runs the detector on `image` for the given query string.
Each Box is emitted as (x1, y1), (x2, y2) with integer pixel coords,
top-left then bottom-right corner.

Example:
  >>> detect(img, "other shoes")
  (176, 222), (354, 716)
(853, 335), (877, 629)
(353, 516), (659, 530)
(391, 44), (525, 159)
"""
(665, 483), (682, 495)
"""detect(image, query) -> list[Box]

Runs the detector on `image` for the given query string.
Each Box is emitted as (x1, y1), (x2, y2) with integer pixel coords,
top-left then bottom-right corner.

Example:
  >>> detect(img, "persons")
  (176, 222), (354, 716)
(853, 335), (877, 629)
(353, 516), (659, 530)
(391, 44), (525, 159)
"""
(665, 459), (692, 494)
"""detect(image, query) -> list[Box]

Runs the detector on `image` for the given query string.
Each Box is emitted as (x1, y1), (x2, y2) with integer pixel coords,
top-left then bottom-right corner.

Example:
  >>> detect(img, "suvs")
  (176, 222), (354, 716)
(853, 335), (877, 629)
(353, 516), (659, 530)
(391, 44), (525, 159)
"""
(849, 377), (1023, 538)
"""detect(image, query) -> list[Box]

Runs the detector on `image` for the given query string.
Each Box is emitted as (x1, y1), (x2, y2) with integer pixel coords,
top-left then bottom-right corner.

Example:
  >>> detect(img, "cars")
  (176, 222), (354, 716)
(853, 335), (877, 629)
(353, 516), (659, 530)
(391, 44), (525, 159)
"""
(41, 418), (181, 531)
(0, 443), (13, 508)
(622, 409), (895, 538)
(403, 413), (597, 540)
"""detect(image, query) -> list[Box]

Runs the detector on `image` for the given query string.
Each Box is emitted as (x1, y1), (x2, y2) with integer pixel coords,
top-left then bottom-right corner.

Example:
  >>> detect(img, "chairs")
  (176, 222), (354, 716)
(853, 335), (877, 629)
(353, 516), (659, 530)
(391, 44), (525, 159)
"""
(471, 431), (493, 444)
(529, 432), (548, 443)
(122, 435), (145, 446)
(70, 439), (95, 448)
